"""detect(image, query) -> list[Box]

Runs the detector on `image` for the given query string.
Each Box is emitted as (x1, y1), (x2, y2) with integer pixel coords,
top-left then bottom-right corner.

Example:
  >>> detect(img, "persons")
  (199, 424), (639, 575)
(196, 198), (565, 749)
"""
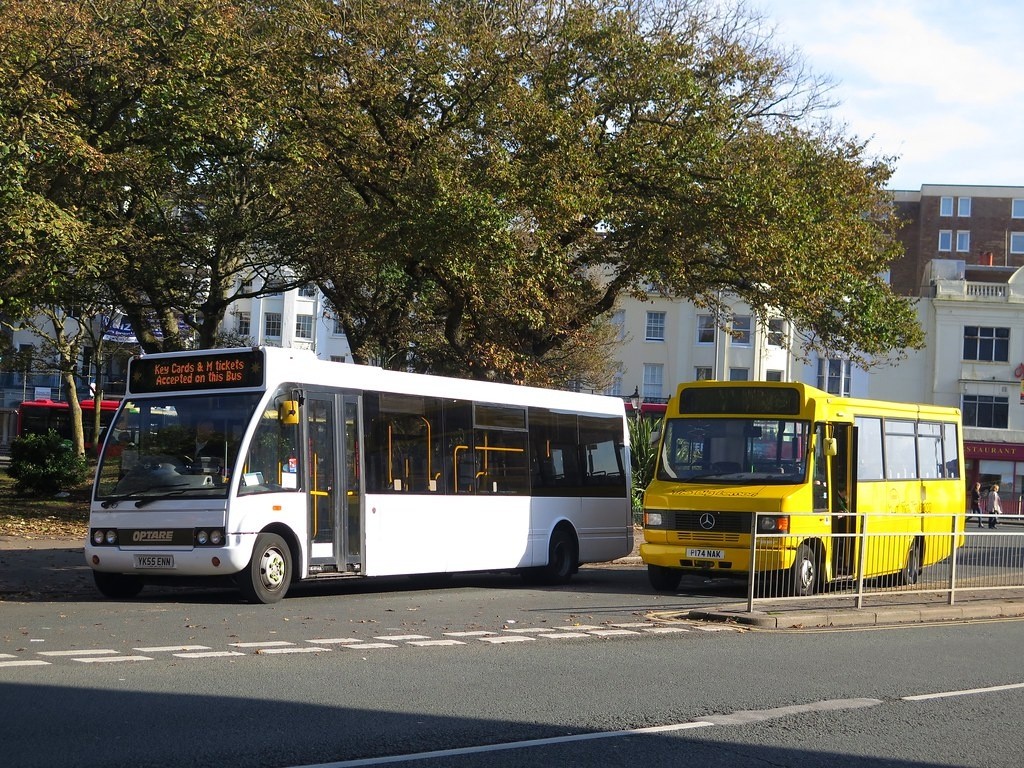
(835, 481), (849, 562)
(181, 417), (223, 469)
(965, 482), (985, 528)
(117, 431), (140, 481)
(987, 485), (1001, 530)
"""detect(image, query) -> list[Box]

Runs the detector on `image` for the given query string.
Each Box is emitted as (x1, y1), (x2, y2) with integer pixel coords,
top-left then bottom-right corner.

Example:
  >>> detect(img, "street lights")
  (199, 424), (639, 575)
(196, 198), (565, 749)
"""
(629, 384), (644, 426)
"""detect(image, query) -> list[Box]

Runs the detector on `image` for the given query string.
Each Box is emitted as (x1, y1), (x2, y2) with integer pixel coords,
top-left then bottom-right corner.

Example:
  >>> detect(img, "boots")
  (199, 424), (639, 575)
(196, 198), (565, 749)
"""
(979, 523), (985, 528)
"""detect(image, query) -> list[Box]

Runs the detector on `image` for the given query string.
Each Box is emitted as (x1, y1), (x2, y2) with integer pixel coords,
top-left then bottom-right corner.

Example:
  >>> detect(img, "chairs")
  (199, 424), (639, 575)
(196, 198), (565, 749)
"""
(490, 469), (624, 492)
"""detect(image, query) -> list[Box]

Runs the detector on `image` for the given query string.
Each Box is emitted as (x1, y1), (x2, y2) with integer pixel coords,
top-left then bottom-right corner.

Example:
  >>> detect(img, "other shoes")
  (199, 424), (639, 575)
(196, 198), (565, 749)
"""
(989, 526), (998, 529)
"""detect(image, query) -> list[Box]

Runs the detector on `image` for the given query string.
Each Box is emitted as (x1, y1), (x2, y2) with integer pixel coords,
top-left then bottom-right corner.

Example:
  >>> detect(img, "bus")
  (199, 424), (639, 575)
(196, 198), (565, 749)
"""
(639, 380), (966, 595)
(17, 398), (166, 460)
(84, 345), (634, 604)
